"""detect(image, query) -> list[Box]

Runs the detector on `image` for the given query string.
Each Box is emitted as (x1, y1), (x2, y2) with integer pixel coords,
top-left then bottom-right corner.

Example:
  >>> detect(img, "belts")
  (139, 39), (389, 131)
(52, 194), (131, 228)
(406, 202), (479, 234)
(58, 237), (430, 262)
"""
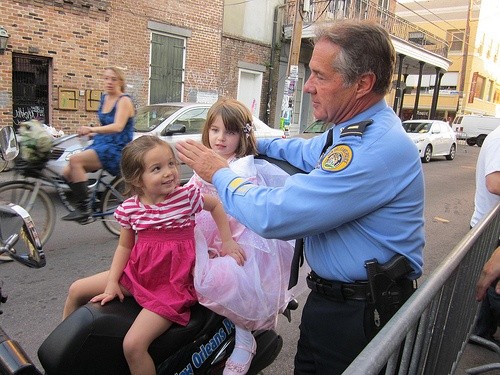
(305, 273), (367, 300)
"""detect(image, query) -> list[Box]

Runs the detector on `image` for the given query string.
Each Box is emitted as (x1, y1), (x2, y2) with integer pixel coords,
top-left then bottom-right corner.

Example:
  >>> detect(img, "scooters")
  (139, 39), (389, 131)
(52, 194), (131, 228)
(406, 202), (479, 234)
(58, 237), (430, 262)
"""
(0, 124), (304, 375)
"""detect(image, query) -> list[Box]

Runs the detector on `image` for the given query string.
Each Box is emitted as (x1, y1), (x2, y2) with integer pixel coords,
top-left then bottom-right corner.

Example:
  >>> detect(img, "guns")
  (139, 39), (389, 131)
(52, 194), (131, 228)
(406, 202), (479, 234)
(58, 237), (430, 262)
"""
(364, 254), (412, 303)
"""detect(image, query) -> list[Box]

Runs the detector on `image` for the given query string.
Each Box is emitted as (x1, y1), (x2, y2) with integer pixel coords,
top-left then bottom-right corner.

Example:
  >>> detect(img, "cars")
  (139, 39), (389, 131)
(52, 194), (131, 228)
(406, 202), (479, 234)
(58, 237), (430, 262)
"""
(401, 119), (457, 163)
(43, 101), (287, 193)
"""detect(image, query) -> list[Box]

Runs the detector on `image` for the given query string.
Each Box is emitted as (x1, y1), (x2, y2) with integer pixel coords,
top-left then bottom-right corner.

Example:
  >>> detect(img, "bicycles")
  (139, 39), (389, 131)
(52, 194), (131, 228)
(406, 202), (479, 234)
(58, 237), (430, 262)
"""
(0, 111), (137, 262)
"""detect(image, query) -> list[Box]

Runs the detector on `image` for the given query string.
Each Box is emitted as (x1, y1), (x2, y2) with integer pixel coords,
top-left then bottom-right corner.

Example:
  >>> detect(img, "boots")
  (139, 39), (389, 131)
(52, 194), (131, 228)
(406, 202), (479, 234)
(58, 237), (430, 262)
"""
(61, 181), (91, 220)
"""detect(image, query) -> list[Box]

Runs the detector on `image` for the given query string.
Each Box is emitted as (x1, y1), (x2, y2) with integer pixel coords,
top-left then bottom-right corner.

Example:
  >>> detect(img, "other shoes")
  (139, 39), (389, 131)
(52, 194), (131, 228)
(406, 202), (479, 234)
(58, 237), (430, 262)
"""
(223, 333), (257, 375)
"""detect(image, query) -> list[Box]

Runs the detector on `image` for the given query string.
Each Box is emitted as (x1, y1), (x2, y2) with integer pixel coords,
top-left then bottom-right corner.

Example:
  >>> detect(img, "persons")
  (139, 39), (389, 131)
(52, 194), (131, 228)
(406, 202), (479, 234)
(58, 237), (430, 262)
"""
(60, 66), (136, 222)
(63, 135), (247, 375)
(184, 97), (295, 375)
(474, 246), (500, 302)
(174, 18), (426, 375)
(470, 124), (500, 247)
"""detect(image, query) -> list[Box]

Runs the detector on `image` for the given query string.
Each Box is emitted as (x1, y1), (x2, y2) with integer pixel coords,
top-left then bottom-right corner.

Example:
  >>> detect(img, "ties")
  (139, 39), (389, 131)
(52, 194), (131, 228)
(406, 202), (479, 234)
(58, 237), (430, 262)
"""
(286, 130), (333, 290)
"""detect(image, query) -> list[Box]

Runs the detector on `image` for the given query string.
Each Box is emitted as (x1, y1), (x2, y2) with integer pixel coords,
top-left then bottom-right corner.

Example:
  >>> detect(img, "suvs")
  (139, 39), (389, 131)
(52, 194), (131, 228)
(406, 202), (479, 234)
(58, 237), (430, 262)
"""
(452, 114), (500, 147)
